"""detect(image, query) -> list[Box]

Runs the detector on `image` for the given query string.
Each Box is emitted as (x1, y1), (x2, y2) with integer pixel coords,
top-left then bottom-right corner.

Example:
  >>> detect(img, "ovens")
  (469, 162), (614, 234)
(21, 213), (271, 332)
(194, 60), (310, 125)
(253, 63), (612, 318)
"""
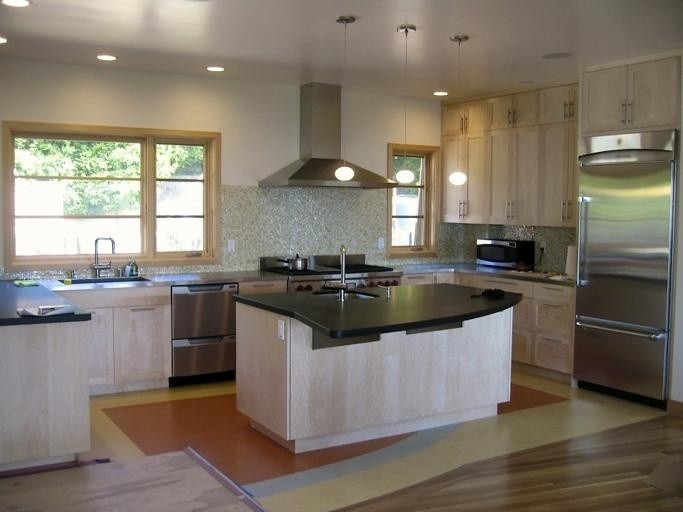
(168, 282), (241, 380)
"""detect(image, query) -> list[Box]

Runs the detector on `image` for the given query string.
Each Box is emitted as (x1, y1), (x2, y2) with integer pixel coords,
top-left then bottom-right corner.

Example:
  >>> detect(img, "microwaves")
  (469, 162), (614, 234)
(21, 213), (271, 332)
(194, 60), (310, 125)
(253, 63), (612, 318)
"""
(473, 236), (536, 274)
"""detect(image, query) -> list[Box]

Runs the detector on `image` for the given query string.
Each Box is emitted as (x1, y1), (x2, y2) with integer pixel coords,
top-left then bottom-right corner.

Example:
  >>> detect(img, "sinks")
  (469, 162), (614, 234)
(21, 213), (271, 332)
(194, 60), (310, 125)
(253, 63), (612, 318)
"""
(57, 275), (153, 286)
(310, 288), (379, 303)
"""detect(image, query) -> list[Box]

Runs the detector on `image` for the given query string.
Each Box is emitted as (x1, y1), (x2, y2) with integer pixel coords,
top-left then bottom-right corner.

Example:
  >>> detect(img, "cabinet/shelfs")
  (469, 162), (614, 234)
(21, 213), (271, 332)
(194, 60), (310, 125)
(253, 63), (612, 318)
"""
(54, 288), (173, 397)
(440, 95), (489, 225)
(485, 87), (539, 227)
(454, 274), (530, 385)
(532, 283), (575, 377)
(401, 274), (453, 287)
(241, 280), (287, 292)
(534, 80), (579, 229)
(579, 54), (682, 132)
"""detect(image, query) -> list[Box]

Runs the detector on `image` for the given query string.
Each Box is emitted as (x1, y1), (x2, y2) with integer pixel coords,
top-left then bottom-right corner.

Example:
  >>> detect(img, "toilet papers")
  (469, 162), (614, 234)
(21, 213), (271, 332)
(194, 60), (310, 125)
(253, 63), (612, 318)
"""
(564, 243), (578, 279)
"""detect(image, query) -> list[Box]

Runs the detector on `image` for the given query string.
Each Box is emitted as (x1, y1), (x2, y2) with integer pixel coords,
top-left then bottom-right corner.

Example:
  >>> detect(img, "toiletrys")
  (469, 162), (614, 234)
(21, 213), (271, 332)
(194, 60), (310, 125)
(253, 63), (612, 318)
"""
(130, 259), (138, 276)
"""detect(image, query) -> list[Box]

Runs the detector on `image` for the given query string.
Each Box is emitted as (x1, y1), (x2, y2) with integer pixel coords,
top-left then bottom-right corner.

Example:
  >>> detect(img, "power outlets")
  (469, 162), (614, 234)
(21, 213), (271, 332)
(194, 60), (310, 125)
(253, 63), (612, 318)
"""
(539, 240), (546, 254)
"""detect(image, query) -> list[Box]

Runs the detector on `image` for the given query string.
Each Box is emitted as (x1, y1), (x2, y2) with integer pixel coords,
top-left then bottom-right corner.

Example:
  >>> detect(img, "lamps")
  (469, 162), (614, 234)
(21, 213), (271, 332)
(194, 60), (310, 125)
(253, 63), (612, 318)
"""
(448, 35), (467, 185)
(395, 23), (414, 184)
(334, 14), (355, 183)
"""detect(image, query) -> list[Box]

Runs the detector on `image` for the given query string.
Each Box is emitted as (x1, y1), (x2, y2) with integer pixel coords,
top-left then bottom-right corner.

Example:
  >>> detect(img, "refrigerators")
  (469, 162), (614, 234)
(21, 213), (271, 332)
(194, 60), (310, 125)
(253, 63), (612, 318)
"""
(569, 126), (679, 417)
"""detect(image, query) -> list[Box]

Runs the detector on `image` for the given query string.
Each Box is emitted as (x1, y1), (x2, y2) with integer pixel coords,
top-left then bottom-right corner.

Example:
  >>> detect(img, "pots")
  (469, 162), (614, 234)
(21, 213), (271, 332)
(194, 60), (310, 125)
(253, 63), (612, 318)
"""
(275, 252), (309, 272)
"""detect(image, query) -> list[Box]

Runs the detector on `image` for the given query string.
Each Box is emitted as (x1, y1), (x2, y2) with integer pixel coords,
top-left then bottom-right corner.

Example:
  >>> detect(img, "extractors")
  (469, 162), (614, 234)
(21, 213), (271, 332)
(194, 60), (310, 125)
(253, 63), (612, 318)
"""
(258, 80), (399, 190)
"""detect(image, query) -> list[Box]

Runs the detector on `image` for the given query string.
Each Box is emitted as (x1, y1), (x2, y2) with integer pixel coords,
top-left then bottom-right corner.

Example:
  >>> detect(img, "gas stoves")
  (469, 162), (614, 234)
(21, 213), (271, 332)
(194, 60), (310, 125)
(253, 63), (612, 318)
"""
(261, 264), (403, 290)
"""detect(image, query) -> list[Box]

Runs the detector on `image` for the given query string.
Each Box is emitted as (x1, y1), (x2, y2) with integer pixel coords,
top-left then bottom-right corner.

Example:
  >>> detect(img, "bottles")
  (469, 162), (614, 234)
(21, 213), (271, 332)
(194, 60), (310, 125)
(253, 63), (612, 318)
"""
(129, 257), (138, 275)
(124, 263), (130, 276)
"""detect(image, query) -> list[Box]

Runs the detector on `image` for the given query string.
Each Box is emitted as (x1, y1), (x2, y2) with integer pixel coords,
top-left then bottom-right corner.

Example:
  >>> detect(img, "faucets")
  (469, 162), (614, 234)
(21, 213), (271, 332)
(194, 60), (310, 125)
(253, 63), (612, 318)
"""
(93, 235), (116, 277)
(336, 241), (348, 302)
